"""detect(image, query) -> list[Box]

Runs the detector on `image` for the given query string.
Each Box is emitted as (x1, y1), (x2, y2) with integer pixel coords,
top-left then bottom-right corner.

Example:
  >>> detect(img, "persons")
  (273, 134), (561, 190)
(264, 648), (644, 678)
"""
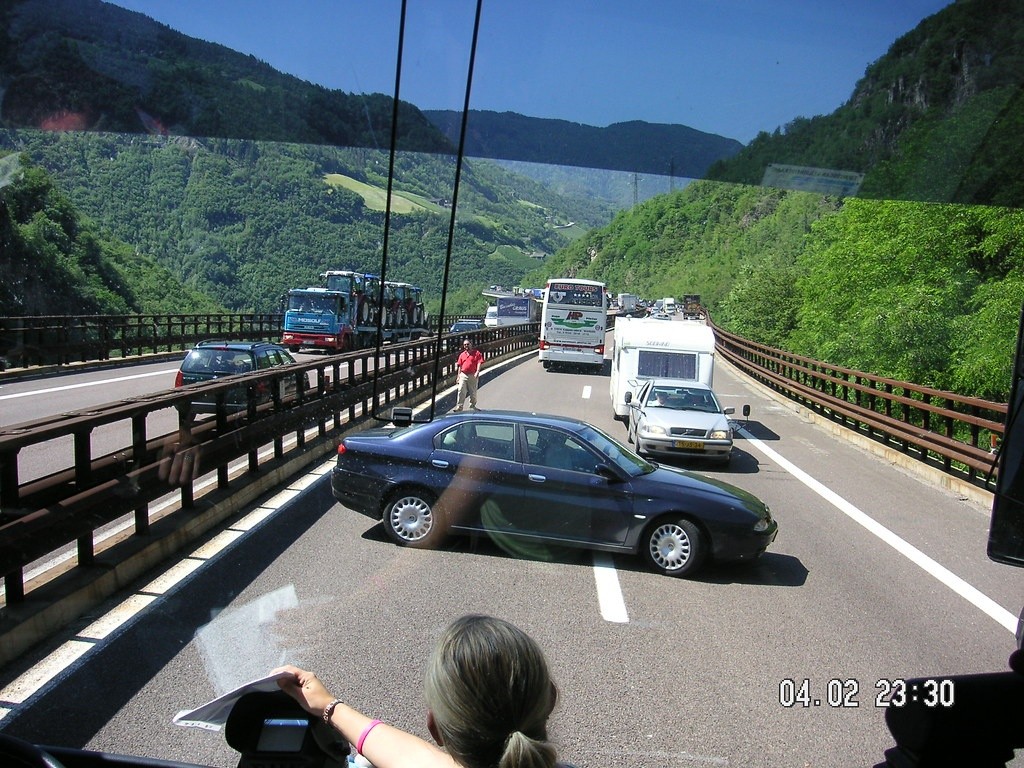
(270, 615), (559, 768)
(453, 340), (485, 412)
(681, 394), (706, 406)
(652, 391), (671, 406)
(544, 433), (590, 471)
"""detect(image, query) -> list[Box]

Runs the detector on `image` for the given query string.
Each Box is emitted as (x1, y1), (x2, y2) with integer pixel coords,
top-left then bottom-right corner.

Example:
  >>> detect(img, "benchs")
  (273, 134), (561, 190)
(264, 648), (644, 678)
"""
(443, 425), (477, 454)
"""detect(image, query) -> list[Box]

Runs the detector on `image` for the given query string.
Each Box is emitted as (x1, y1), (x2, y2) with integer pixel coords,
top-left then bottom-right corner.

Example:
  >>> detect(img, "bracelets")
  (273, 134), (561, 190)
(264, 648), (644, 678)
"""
(357, 720), (384, 756)
(322, 699), (344, 725)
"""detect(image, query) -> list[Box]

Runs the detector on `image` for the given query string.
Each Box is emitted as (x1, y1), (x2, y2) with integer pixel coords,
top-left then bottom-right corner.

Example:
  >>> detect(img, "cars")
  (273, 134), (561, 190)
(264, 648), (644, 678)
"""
(647, 306), (671, 320)
(331, 410), (778, 579)
(637, 298), (663, 310)
(625, 378), (735, 463)
(449, 319), (487, 345)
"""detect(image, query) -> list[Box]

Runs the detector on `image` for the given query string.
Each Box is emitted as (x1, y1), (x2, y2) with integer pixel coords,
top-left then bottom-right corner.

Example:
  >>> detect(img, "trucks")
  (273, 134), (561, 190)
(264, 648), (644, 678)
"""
(609, 315), (716, 421)
(683, 294), (701, 319)
(532, 288), (545, 299)
(617, 293), (637, 312)
(663, 297), (675, 314)
(495, 297), (539, 330)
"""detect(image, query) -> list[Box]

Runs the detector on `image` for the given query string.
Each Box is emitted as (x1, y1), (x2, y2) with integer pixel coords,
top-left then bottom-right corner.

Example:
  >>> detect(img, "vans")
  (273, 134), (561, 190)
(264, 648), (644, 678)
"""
(484, 306), (498, 328)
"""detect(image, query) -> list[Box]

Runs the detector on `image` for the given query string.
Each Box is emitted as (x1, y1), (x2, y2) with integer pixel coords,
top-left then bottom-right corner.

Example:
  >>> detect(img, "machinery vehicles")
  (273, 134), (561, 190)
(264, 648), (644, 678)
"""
(320, 271), (425, 327)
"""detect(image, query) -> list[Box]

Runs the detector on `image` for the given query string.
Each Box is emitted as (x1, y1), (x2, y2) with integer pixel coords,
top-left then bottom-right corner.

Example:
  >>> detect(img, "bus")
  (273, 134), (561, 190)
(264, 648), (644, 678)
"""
(539, 279), (612, 371)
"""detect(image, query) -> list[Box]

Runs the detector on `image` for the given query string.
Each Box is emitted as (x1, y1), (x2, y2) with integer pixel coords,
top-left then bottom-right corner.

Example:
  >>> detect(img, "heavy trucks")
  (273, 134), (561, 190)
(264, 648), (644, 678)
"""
(283, 288), (429, 354)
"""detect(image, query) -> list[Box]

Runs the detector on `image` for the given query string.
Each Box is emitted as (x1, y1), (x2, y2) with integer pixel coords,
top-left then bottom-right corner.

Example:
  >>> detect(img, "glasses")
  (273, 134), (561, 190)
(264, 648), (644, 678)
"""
(464, 344), (469, 346)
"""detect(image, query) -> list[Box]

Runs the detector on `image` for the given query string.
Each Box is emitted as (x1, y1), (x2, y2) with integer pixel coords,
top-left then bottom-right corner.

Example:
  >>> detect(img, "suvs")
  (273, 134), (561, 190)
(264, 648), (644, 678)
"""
(174, 339), (310, 424)
